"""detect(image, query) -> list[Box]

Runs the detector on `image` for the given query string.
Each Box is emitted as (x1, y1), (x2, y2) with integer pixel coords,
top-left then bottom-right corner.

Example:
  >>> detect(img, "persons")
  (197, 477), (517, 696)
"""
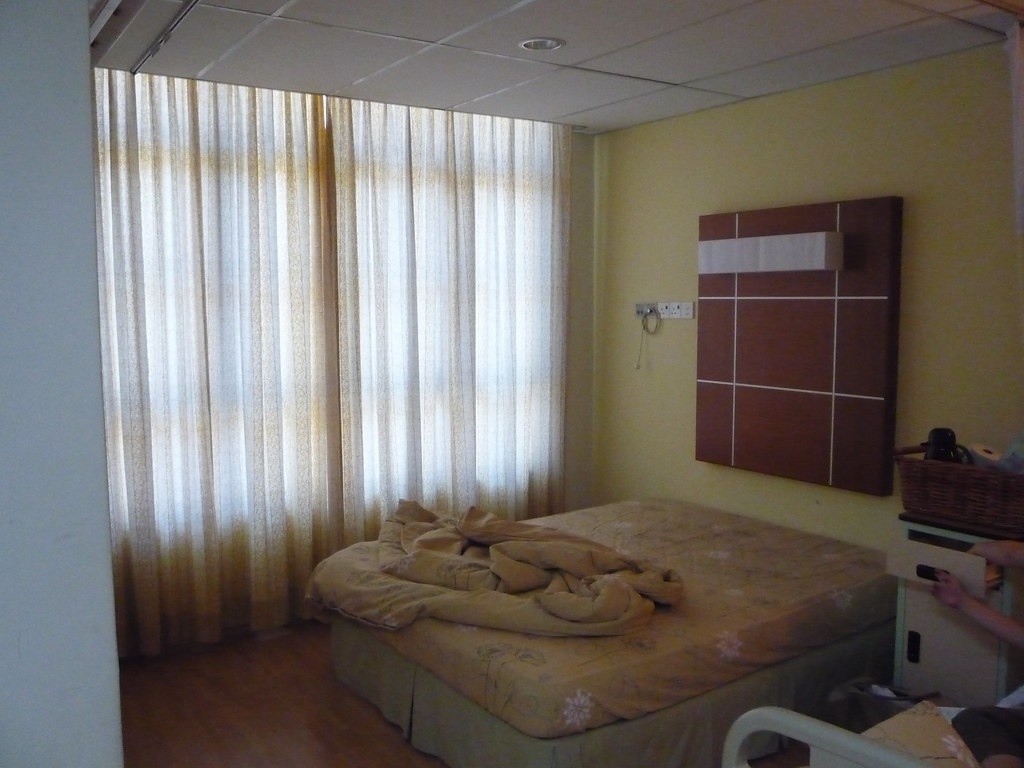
(928, 540), (1024, 768)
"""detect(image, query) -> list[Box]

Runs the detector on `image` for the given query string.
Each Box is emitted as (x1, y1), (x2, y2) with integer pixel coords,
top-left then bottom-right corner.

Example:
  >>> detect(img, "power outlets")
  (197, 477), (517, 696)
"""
(634, 301), (680, 320)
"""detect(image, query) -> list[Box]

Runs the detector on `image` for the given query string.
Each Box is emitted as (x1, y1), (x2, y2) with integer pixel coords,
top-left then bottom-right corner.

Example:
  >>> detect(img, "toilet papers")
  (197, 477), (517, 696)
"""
(962, 442), (1001, 467)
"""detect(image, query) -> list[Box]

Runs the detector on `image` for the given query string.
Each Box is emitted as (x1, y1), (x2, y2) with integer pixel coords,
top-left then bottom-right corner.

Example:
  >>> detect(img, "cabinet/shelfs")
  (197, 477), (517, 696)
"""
(886, 510), (1016, 706)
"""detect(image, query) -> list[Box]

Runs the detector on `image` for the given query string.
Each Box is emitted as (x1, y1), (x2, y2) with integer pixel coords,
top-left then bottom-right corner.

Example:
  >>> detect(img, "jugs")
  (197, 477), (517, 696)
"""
(921, 428), (973, 465)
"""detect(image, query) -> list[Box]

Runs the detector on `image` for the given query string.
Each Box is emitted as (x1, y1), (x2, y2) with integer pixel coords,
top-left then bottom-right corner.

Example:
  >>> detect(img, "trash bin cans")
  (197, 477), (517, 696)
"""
(840, 676), (941, 731)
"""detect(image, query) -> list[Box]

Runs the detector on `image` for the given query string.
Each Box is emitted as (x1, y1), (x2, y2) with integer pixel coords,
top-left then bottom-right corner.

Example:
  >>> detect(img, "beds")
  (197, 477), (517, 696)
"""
(302, 497), (898, 767)
(722, 664), (1024, 768)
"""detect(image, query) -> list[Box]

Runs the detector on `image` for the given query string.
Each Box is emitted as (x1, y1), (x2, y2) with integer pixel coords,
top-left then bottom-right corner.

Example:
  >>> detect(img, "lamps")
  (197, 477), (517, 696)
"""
(694, 232), (846, 273)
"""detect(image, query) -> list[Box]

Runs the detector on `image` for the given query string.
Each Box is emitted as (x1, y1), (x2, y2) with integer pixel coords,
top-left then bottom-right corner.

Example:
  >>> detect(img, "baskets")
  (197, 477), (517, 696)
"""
(892, 444), (1024, 535)
